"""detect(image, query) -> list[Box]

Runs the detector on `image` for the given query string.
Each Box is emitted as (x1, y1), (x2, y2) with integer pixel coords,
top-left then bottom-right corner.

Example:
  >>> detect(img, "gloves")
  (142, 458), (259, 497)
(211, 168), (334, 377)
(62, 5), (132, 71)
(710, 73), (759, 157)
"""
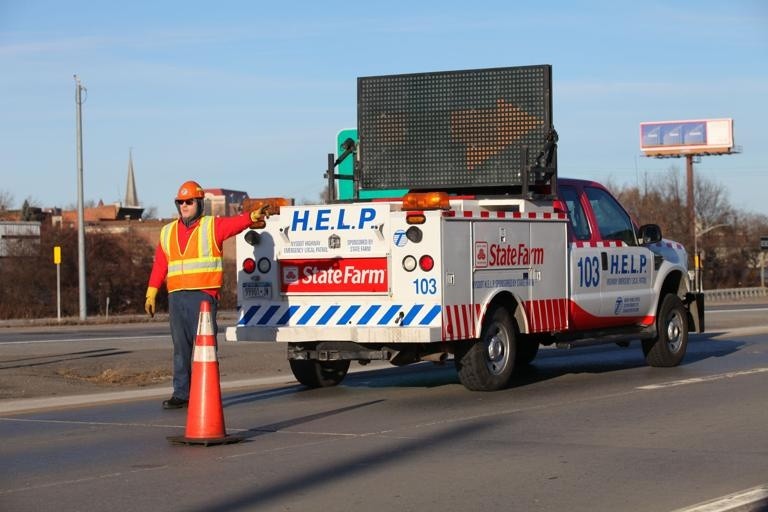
(144, 286), (158, 318)
(249, 203), (271, 223)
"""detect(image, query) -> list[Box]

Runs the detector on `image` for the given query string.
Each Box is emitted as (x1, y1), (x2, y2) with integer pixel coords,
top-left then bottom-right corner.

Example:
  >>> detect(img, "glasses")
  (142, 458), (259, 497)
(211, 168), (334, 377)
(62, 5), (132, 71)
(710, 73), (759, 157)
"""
(177, 199), (194, 206)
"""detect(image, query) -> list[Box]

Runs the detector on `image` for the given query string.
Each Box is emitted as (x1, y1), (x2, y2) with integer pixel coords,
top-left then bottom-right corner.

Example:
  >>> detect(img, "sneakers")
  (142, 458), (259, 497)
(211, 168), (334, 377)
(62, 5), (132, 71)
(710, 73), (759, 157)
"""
(160, 394), (187, 410)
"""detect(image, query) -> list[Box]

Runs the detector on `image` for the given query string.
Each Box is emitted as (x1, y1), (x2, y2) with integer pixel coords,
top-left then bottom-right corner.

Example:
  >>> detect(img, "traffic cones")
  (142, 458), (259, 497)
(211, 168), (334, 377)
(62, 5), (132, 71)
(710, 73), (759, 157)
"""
(166, 300), (242, 447)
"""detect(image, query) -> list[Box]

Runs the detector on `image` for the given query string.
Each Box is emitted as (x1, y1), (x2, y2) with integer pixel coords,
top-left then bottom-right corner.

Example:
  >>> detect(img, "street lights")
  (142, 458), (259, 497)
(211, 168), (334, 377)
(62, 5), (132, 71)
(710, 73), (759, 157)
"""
(71, 72), (87, 320)
(693, 224), (734, 293)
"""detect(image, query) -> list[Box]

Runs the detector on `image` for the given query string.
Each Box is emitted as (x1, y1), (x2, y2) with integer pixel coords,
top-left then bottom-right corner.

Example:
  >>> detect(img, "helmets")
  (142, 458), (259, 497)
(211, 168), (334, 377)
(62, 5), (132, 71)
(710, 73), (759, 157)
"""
(173, 178), (206, 202)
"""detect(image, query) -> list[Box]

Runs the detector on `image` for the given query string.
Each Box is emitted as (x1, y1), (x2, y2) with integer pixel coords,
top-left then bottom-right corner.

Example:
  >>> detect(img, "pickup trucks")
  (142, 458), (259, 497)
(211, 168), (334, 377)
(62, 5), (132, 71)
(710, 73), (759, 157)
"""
(226, 65), (693, 389)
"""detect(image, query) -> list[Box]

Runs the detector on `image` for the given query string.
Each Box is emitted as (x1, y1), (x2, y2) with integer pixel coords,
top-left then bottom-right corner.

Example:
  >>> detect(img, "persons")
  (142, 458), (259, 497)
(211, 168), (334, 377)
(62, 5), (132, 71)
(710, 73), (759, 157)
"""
(144, 181), (269, 408)
